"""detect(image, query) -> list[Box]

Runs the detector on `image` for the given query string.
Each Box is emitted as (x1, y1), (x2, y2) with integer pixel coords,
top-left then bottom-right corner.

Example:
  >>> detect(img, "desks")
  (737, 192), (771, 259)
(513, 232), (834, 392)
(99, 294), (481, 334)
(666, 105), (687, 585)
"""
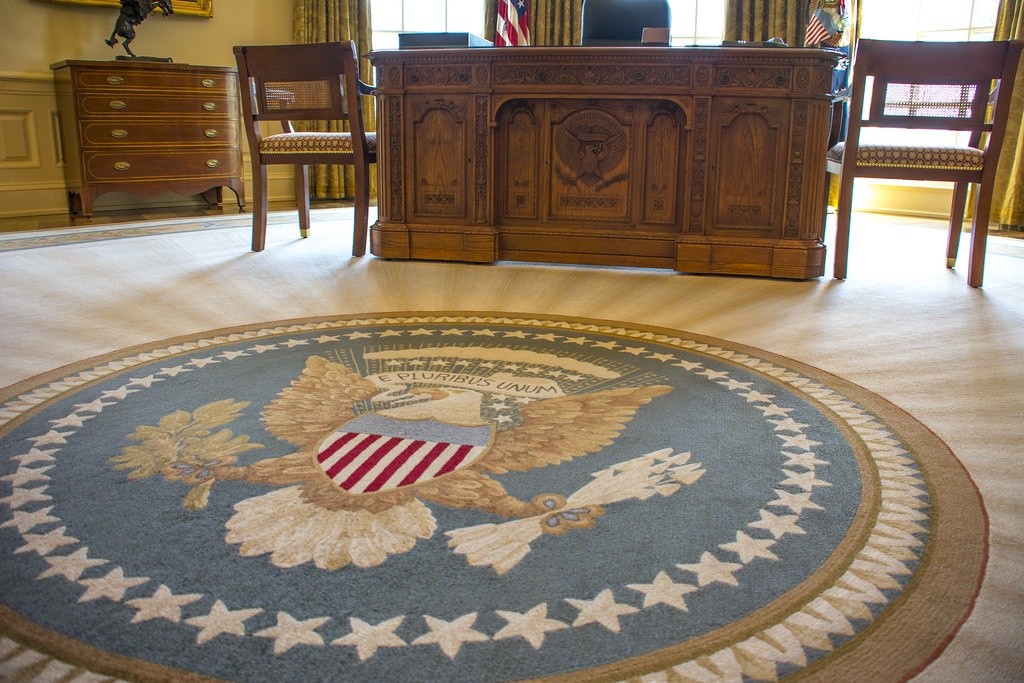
(49, 59), (245, 217)
(362, 48), (847, 279)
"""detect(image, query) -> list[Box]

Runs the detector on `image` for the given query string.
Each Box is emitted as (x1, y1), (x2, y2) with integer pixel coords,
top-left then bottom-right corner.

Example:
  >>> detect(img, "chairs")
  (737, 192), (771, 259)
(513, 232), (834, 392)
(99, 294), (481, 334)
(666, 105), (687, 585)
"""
(823, 38), (1024, 287)
(579, 0), (670, 47)
(233, 40), (377, 256)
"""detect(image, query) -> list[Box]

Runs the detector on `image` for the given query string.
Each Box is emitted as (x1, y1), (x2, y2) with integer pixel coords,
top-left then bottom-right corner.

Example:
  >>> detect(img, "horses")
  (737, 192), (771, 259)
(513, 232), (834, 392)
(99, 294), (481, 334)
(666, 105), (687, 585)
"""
(104, 0), (174, 58)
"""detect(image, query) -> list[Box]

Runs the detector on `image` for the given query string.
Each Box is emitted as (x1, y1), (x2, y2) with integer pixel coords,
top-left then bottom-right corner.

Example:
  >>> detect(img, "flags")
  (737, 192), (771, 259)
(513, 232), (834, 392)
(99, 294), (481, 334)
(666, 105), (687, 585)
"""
(496, 1), (532, 48)
(804, 6), (839, 47)
